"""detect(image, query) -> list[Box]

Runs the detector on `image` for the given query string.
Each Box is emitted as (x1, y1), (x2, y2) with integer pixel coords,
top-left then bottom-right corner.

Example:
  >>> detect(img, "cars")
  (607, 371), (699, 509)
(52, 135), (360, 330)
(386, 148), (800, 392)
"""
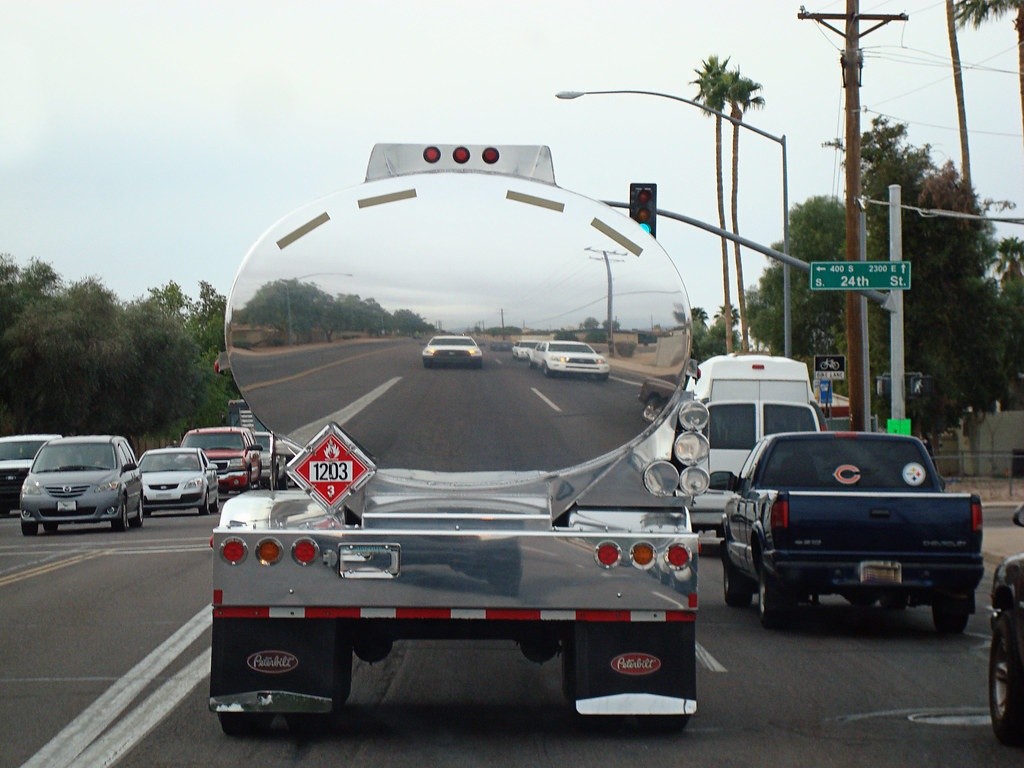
(0, 435), (65, 518)
(422, 334), (484, 371)
(986, 497), (1024, 746)
(20, 434), (143, 536)
(253, 431), (290, 491)
(512, 339), (539, 360)
(137, 447), (220, 516)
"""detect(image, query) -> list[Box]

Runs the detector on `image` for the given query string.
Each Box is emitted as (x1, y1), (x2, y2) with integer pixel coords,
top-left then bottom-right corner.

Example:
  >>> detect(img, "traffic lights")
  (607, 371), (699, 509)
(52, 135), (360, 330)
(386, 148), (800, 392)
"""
(912, 376), (934, 396)
(629, 182), (657, 239)
(875, 376), (891, 397)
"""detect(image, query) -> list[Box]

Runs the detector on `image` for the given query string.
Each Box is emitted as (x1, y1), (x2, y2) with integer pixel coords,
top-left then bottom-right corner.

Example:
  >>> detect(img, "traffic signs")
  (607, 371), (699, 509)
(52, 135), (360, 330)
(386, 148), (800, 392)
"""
(809, 262), (911, 288)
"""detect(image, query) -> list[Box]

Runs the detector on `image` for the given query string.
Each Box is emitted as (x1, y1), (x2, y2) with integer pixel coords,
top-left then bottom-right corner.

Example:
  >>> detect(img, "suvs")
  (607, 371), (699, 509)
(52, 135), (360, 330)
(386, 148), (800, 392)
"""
(181, 426), (263, 494)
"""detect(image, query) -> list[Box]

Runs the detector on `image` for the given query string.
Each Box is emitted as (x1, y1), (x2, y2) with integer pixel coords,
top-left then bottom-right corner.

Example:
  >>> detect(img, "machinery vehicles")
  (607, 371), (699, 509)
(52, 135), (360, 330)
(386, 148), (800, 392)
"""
(209, 139), (711, 736)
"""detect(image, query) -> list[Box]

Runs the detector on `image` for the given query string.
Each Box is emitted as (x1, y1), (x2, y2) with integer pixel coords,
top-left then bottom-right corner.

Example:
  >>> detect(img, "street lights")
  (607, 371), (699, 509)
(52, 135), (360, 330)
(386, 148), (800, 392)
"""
(555, 89), (793, 360)
(286, 272), (354, 348)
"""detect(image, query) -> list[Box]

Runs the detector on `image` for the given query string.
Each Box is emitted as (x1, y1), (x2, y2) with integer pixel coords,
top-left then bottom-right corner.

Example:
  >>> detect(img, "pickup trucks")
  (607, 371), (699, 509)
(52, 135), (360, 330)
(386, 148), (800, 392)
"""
(528, 341), (612, 382)
(708, 428), (988, 635)
(638, 373), (677, 420)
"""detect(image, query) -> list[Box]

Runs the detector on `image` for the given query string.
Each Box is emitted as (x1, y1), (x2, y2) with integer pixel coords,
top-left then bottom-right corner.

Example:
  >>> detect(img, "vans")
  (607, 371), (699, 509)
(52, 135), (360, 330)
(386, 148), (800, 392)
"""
(684, 353), (814, 404)
(686, 399), (823, 535)
